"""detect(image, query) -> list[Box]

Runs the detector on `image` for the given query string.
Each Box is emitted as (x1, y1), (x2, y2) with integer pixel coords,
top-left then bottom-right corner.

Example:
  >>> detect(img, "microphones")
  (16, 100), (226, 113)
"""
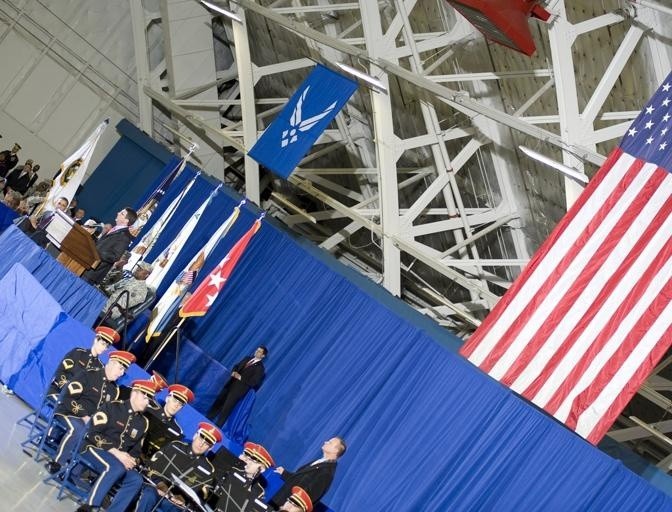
(88, 223), (104, 229)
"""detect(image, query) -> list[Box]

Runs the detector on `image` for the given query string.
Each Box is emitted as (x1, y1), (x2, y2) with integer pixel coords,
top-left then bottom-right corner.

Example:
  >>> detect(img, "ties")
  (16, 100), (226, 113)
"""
(107, 227), (116, 237)
(39, 214), (55, 231)
(244, 360), (256, 370)
(73, 217), (78, 222)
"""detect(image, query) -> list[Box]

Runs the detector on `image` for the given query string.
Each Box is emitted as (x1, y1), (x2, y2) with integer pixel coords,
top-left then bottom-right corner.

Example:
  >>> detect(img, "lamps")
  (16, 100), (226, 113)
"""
(450, 1), (551, 58)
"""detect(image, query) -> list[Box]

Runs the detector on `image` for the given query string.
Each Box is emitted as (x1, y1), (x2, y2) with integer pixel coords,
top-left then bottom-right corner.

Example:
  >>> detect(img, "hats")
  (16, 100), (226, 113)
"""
(131, 380), (156, 401)
(197, 421), (312, 512)
(109, 351), (136, 368)
(137, 261), (153, 273)
(95, 326), (120, 345)
(168, 384), (194, 403)
(15, 142), (21, 149)
(150, 370), (169, 392)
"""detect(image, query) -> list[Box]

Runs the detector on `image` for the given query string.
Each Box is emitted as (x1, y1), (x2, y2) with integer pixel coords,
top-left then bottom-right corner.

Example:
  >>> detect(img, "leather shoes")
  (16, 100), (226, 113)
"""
(36, 434), (101, 511)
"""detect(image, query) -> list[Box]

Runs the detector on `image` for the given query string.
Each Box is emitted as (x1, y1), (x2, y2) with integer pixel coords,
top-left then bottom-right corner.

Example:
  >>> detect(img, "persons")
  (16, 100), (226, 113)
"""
(0, 133), (153, 319)
(0, 326), (347, 512)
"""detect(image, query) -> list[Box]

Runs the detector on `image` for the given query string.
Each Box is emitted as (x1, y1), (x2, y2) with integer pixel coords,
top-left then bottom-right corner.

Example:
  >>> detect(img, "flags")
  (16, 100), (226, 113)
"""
(125, 146), (271, 343)
(247, 65), (360, 179)
(34, 119), (109, 213)
(457, 70), (672, 446)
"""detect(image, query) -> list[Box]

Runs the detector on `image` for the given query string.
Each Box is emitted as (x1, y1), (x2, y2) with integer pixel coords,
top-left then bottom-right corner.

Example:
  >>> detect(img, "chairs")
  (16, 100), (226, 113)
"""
(18, 376), (101, 512)
(95, 269), (156, 351)
